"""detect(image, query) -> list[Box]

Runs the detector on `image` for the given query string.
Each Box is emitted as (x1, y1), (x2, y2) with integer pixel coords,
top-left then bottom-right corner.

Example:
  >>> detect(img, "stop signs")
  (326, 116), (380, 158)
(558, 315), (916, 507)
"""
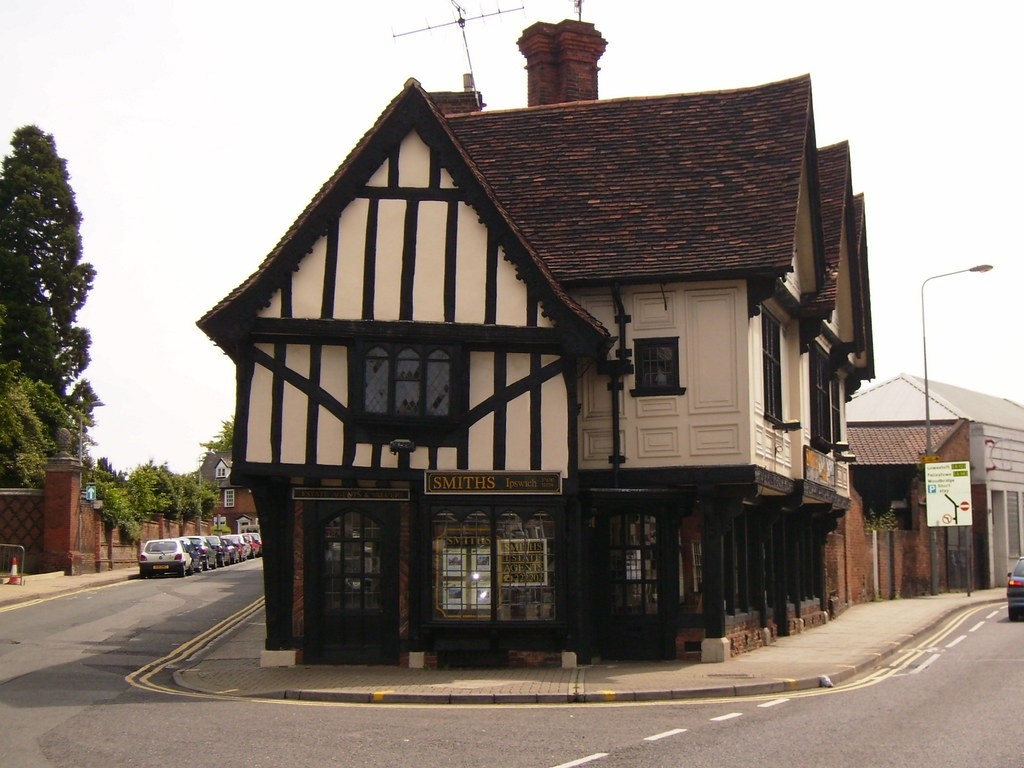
(960, 502), (969, 511)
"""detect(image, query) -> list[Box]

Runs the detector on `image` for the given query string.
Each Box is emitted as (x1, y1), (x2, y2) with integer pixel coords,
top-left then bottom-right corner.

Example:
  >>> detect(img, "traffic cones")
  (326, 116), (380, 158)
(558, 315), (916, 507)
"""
(6, 557), (20, 585)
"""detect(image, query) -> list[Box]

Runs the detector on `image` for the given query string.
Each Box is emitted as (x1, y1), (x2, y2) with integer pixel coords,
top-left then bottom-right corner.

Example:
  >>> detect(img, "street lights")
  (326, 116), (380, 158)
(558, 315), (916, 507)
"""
(198, 451), (214, 536)
(78, 401), (106, 575)
(921, 265), (995, 596)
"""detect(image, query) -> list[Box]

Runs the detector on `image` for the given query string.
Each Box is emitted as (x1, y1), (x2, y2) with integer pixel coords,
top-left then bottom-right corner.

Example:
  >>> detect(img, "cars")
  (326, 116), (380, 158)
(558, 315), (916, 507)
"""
(1006, 557), (1024, 622)
(240, 534), (262, 558)
(205, 536), (231, 567)
(242, 533), (262, 555)
(183, 536), (218, 570)
(178, 537), (204, 573)
(220, 536), (241, 564)
(224, 535), (244, 561)
(232, 536), (253, 559)
(139, 539), (192, 578)
